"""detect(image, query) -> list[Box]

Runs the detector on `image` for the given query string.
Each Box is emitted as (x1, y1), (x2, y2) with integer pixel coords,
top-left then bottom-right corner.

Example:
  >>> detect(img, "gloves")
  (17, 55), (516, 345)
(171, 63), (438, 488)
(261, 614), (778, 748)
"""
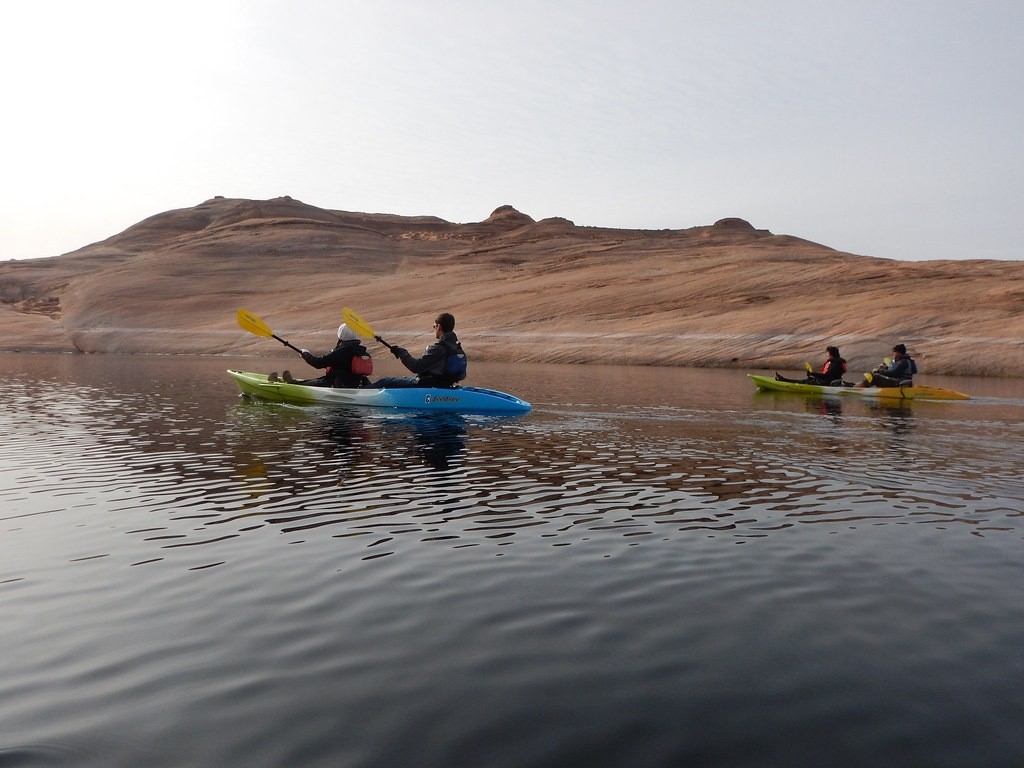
(298, 348), (310, 358)
(390, 346), (403, 359)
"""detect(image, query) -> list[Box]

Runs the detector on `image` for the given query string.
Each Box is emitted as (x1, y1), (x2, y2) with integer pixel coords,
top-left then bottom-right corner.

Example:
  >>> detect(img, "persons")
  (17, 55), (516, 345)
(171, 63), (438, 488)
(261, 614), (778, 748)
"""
(358, 312), (459, 388)
(775, 345), (847, 387)
(843, 343), (913, 388)
(267, 322), (367, 389)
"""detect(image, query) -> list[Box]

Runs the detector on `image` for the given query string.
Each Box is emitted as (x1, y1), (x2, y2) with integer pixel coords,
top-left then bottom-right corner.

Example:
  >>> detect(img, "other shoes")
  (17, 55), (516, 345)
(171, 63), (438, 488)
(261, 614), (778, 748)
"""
(350, 380), (360, 388)
(843, 381), (855, 387)
(360, 377), (371, 386)
(283, 371), (293, 384)
(268, 372), (277, 382)
(775, 371), (784, 381)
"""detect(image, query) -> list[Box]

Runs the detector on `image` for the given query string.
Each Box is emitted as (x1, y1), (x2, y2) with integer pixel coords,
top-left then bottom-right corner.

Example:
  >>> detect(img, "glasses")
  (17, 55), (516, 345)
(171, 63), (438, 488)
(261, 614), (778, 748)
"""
(433, 324), (442, 329)
(892, 350), (896, 353)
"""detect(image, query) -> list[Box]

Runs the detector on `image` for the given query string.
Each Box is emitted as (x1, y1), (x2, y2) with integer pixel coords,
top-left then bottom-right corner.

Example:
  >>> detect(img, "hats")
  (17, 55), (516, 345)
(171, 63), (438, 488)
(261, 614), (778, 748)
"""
(336, 323), (356, 341)
(893, 344), (906, 354)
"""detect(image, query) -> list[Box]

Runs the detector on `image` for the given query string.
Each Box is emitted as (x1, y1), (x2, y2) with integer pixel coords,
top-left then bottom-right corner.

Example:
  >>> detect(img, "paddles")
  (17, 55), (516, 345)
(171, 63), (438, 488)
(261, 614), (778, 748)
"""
(863, 356), (891, 383)
(236, 308), (302, 354)
(805, 362), (813, 373)
(341, 305), (391, 349)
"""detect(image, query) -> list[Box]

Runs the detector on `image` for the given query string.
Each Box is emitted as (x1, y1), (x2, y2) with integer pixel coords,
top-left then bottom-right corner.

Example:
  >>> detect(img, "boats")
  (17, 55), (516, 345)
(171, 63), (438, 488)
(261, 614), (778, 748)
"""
(227, 369), (532, 413)
(746, 373), (975, 401)
